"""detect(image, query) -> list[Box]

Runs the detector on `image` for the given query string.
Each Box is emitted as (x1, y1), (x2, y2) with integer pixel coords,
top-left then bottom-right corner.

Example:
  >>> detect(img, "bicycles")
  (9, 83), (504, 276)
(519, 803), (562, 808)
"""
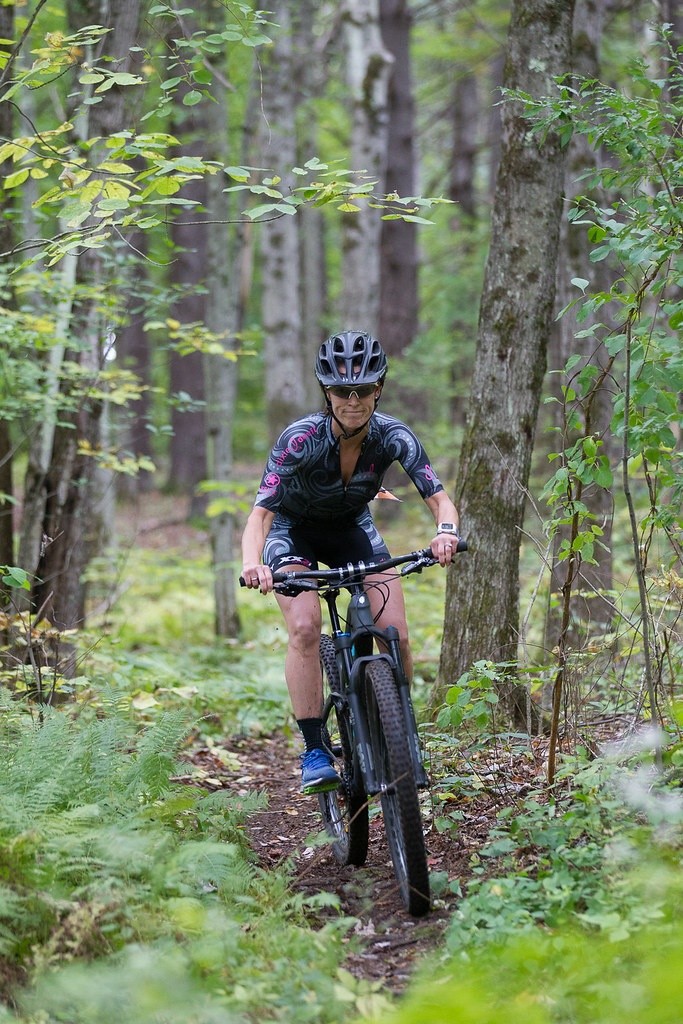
(240, 538), (472, 916)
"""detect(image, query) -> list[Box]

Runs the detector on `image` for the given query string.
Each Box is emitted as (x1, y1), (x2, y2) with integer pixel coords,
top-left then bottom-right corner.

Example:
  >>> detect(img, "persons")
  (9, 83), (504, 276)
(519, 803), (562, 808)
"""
(239, 328), (460, 795)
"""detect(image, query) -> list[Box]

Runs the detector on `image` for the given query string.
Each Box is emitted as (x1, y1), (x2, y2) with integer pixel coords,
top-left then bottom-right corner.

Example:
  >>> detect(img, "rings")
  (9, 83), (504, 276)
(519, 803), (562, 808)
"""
(444, 543), (452, 547)
(251, 577), (257, 580)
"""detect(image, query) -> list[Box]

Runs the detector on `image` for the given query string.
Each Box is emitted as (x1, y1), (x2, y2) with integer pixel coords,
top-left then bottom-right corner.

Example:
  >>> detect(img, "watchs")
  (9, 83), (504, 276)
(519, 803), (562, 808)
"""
(437, 522), (459, 537)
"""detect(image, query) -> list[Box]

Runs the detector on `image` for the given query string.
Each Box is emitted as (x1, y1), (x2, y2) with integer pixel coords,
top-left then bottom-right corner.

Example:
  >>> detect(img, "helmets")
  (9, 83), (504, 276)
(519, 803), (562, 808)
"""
(314, 330), (388, 385)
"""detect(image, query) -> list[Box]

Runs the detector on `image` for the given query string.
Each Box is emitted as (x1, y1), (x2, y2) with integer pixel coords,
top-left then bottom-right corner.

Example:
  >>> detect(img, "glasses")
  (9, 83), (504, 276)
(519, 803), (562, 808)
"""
(323, 380), (380, 399)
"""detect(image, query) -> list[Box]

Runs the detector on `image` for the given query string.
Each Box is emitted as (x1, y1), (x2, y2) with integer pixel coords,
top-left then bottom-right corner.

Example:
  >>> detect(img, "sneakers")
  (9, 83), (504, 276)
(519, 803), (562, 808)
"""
(299, 749), (343, 788)
(413, 761), (430, 788)
